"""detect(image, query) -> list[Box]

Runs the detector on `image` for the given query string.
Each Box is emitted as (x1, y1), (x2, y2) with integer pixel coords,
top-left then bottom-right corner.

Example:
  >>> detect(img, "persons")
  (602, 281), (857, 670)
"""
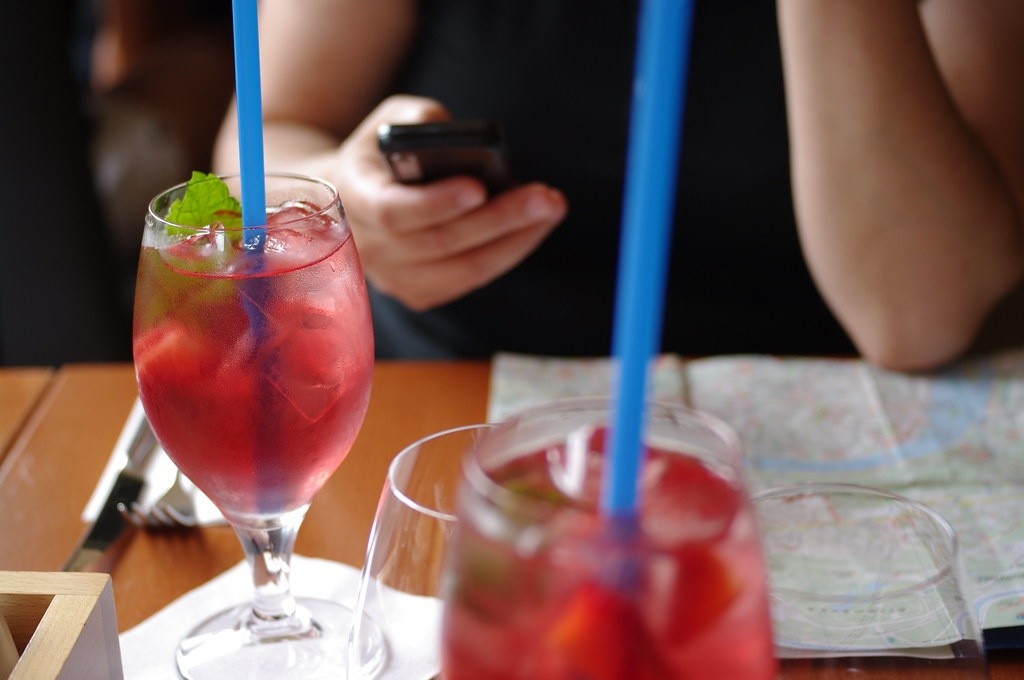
(213, 1), (1022, 371)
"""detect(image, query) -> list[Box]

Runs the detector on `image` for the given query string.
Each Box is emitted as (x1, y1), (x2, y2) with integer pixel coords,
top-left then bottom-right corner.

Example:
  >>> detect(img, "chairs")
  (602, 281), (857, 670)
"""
(84, 1), (245, 250)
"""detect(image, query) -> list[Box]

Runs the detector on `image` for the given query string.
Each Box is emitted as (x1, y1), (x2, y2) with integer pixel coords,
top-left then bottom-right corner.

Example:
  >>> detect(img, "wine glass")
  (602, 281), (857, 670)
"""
(748, 480), (988, 679)
(135, 174), (394, 678)
(443, 399), (779, 679)
(350, 423), (495, 679)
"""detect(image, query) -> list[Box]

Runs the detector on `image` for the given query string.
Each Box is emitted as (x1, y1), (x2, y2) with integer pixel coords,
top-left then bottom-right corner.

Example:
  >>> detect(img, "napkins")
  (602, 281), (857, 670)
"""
(111, 552), (449, 680)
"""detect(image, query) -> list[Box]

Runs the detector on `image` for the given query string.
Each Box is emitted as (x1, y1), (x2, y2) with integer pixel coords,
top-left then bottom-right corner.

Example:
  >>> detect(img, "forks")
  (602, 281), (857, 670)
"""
(121, 466), (199, 527)
(58, 417), (159, 573)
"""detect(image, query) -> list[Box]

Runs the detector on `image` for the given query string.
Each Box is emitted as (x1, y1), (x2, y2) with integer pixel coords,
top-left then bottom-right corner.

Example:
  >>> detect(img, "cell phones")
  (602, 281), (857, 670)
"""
(377, 122), (511, 198)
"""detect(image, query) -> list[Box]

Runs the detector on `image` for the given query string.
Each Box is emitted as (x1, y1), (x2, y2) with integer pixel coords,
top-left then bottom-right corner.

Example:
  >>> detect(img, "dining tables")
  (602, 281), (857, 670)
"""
(5, 363), (1023, 680)
